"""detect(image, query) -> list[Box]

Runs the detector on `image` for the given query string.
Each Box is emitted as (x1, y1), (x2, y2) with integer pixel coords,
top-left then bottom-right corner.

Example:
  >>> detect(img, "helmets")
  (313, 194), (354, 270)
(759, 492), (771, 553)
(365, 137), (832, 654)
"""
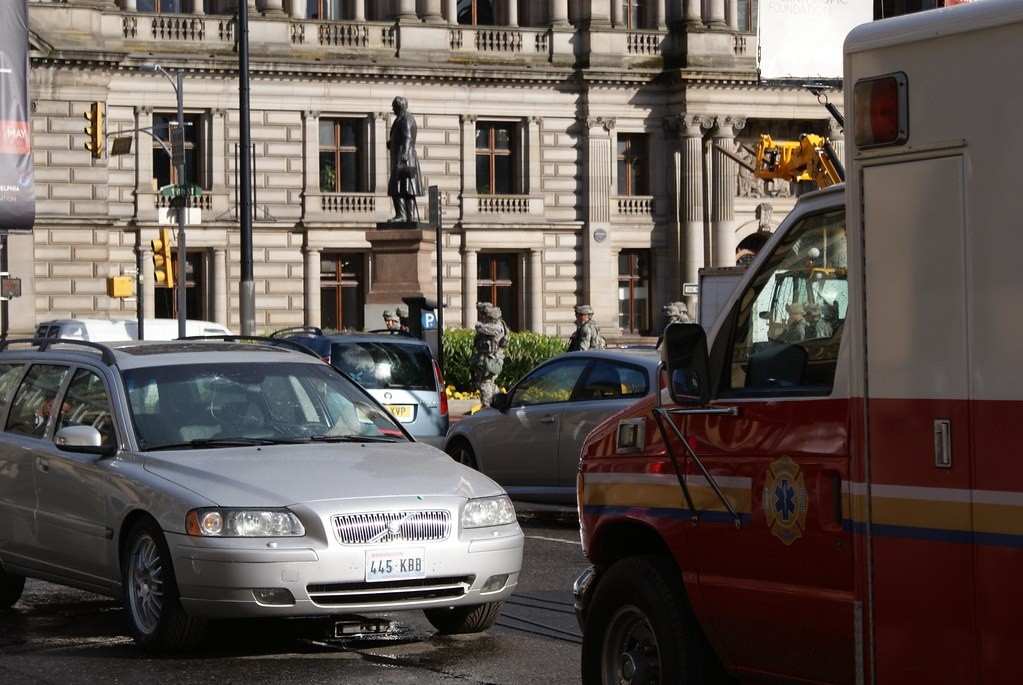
(805, 303), (821, 316)
(397, 307), (410, 317)
(786, 303), (803, 314)
(477, 302), (493, 312)
(484, 307), (502, 319)
(383, 311), (399, 321)
(663, 306), (679, 316)
(670, 301), (687, 312)
(576, 305), (593, 314)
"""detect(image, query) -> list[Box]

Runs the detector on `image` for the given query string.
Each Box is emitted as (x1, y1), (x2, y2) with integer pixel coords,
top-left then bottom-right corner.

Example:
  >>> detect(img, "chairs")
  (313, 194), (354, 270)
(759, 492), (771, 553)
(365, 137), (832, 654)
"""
(210, 399), (276, 442)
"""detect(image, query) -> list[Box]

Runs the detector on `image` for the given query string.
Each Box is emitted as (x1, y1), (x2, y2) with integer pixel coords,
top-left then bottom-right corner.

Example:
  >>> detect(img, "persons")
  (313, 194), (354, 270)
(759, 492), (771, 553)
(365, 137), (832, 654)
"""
(386, 95), (424, 223)
(20, 394), (72, 436)
(383, 307), (408, 333)
(786, 303), (832, 342)
(470, 302), (508, 407)
(569, 305), (604, 350)
(661, 302), (690, 326)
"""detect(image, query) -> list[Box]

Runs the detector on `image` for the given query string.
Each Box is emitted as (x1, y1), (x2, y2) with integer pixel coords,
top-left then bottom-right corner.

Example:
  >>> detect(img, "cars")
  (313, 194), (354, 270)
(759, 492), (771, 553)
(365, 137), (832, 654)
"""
(30, 320), (239, 346)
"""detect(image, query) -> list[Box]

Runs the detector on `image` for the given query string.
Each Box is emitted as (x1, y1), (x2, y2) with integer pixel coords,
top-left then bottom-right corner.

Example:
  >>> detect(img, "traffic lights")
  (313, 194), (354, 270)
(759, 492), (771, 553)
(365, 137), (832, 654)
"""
(83, 101), (103, 160)
(151, 229), (174, 288)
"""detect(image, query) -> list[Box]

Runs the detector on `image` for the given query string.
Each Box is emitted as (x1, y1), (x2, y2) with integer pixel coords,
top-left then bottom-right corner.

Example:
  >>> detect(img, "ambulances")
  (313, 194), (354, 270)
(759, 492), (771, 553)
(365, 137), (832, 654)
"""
(568, 0), (1023, 685)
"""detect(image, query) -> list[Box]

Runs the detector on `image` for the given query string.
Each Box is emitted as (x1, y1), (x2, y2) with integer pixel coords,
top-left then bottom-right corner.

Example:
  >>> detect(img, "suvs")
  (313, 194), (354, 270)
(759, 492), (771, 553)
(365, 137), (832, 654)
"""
(445, 345), (680, 507)
(0, 337), (524, 657)
(268, 325), (449, 438)
